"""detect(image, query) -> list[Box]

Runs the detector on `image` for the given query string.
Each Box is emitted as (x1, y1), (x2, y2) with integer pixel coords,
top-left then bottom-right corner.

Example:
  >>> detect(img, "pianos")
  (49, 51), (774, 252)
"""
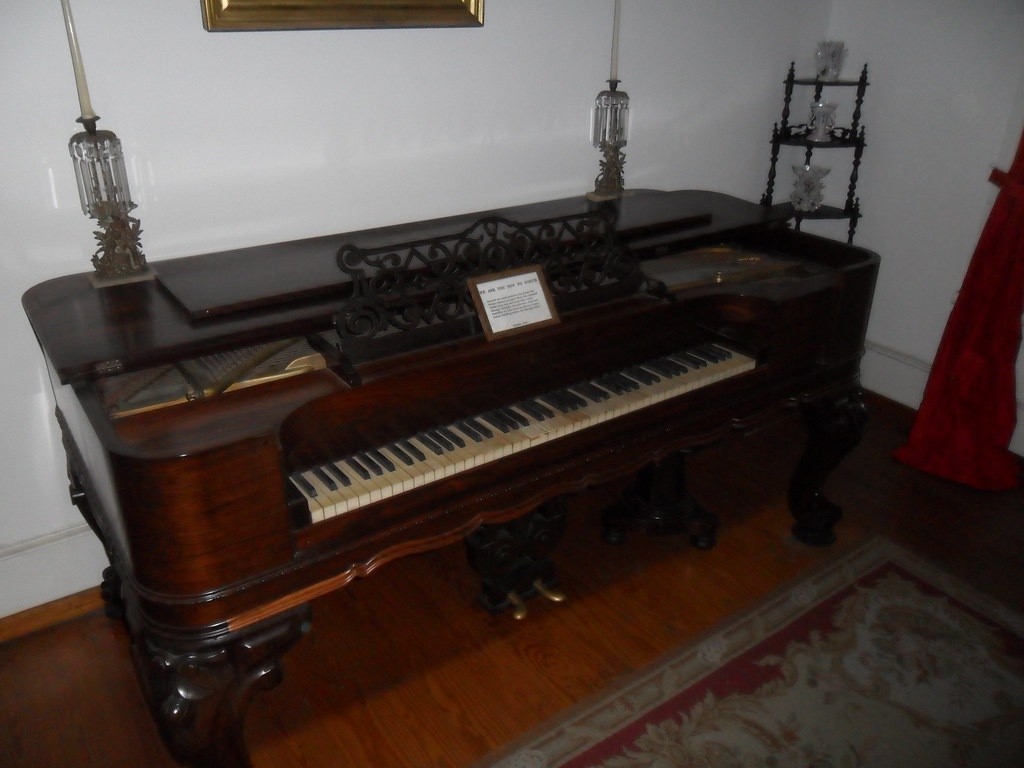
(14, 187), (886, 767)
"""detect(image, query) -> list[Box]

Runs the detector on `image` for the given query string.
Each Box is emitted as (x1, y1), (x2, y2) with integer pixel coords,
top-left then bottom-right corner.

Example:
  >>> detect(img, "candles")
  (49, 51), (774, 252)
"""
(60, 0), (97, 119)
(610, 0), (621, 79)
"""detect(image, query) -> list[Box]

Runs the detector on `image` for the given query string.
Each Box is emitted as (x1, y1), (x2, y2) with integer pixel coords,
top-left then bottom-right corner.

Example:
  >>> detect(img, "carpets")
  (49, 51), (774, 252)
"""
(481, 528), (1024, 768)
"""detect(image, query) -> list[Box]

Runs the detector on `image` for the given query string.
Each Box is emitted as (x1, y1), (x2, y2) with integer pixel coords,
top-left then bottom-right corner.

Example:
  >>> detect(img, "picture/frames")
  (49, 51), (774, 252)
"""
(199, 0), (485, 32)
(465, 265), (562, 344)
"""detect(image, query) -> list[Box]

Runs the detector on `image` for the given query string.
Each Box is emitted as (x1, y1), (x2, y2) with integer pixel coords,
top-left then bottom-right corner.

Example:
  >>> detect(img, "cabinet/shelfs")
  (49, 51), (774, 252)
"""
(760, 60), (871, 244)
